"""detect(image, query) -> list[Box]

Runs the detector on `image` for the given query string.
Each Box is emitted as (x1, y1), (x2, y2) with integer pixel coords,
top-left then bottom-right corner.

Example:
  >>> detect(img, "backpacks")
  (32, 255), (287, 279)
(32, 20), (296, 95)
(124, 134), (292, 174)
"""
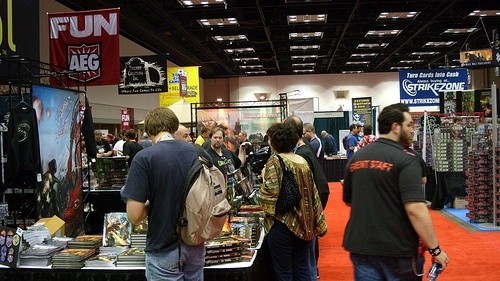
(343, 135), (348, 150)
(172, 144), (231, 246)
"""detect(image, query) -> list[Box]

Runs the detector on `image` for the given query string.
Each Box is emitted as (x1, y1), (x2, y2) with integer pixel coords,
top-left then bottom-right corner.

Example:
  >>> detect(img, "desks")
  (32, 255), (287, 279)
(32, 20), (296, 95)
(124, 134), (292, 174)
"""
(83, 179), (127, 236)
(420, 159), (468, 208)
(0, 216), (267, 281)
(323, 155), (348, 182)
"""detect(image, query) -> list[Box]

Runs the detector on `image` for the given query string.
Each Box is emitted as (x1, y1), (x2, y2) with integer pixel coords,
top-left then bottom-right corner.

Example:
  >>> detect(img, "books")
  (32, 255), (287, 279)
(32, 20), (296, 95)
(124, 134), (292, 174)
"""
(17, 200), (272, 269)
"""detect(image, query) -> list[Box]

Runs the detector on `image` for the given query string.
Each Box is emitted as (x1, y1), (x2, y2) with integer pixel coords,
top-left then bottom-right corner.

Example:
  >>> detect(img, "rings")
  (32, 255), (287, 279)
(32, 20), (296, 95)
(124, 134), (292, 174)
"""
(446, 260), (448, 263)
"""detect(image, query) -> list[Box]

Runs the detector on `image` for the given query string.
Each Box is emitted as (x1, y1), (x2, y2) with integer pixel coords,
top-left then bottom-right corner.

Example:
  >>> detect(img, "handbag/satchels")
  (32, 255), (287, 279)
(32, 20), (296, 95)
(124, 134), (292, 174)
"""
(276, 153), (302, 215)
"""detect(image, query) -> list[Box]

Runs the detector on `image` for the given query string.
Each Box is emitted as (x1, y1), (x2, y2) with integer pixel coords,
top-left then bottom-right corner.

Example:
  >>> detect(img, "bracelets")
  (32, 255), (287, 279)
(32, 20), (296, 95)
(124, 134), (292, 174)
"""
(428, 244), (442, 256)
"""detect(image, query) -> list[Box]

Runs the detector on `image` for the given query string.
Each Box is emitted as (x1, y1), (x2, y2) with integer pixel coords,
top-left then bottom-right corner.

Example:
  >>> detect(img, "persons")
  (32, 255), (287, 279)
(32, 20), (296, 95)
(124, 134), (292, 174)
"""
(434, 104), (493, 133)
(465, 52), (479, 62)
(204, 126), (251, 204)
(339, 102), (450, 281)
(253, 121), (328, 281)
(280, 114), (330, 280)
(119, 106), (214, 281)
(91, 118), (378, 181)
(477, 52), (488, 62)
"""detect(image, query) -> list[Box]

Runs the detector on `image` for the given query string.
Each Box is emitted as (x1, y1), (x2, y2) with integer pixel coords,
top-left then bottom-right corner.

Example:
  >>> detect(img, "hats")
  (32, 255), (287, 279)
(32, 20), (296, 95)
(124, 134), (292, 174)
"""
(356, 120), (364, 128)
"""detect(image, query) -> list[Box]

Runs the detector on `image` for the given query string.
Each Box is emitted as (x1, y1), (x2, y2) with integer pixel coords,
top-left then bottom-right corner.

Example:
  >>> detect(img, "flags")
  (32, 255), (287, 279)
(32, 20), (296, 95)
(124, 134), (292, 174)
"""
(0, 0), (201, 109)
